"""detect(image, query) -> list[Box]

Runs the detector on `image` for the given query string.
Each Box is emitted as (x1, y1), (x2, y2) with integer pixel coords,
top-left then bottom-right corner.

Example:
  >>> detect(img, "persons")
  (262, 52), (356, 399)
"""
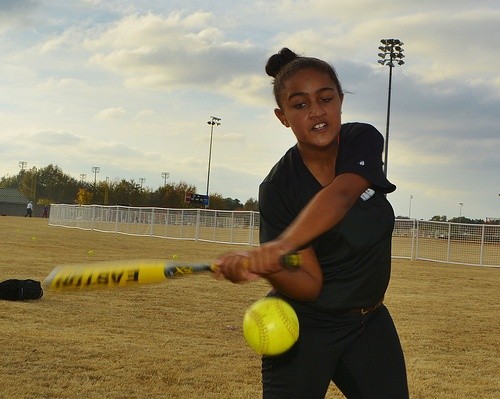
(42, 205), (48, 218)
(210, 46), (410, 399)
(24, 201), (33, 217)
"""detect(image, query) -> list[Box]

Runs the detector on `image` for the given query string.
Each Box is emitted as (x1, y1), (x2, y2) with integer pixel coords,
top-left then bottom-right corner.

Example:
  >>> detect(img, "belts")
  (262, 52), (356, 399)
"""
(353, 298), (384, 316)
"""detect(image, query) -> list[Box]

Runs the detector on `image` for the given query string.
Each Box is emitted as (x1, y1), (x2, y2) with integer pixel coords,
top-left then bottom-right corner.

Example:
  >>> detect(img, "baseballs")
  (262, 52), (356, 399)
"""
(242, 296), (300, 355)
(32, 237), (36, 241)
(173, 255), (178, 260)
(88, 251), (95, 256)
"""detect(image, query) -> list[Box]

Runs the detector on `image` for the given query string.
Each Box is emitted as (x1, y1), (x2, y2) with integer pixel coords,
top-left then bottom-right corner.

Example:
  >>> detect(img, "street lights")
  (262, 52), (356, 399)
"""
(18, 161), (28, 177)
(91, 166), (101, 204)
(80, 173), (87, 186)
(377, 39), (405, 198)
(139, 177), (146, 192)
(205, 115), (222, 209)
(409, 194), (413, 219)
(459, 202), (464, 230)
(161, 171), (170, 184)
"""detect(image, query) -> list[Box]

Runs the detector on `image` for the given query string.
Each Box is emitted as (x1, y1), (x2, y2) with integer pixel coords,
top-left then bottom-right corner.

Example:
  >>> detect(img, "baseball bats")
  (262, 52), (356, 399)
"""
(45, 252), (301, 289)
